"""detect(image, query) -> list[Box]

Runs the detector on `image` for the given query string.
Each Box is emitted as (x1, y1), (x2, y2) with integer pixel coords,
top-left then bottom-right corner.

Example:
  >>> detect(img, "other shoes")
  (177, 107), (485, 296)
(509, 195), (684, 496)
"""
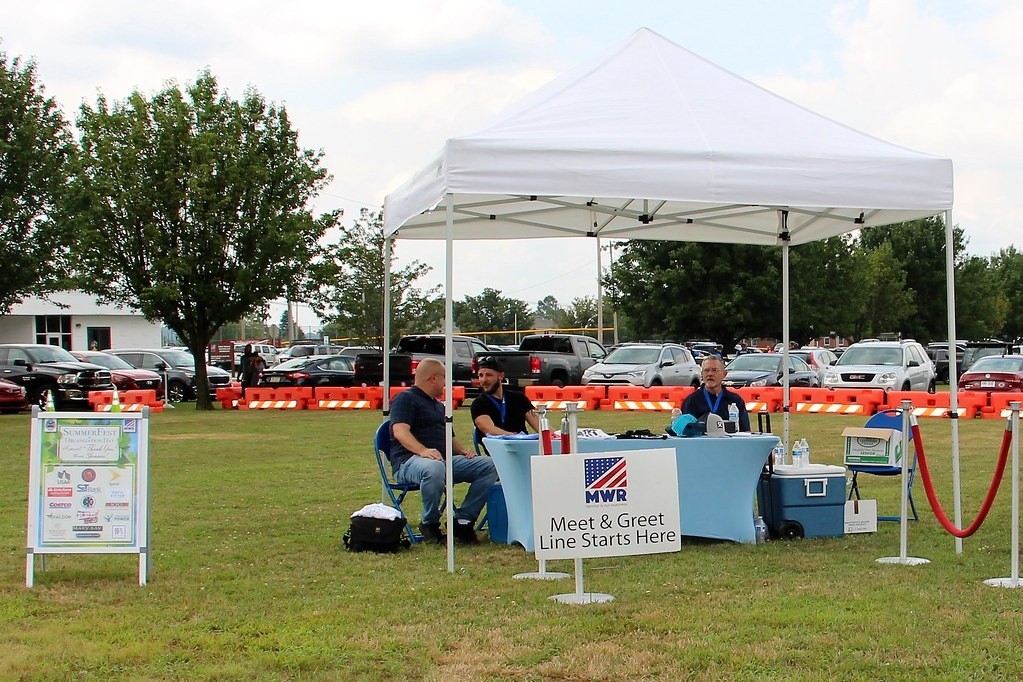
(445, 517), (477, 541)
(419, 521), (447, 546)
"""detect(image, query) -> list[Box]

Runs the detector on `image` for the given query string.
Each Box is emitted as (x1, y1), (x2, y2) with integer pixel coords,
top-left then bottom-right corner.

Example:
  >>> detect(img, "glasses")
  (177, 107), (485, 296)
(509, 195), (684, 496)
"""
(702, 368), (721, 373)
(427, 373), (445, 381)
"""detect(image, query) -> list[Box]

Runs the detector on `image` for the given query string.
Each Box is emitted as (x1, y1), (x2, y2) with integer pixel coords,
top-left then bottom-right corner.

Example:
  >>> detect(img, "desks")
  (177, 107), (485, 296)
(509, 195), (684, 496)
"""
(482, 436), (781, 552)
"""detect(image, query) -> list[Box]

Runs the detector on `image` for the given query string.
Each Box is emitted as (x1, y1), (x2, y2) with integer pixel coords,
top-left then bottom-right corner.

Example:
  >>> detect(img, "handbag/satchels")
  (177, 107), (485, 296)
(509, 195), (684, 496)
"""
(343, 503), (411, 554)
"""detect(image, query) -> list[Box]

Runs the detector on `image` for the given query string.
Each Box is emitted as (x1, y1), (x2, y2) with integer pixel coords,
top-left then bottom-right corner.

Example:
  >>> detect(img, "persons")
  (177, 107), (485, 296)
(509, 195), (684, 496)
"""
(387, 358), (497, 544)
(238, 343), (270, 400)
(736, 343), (751, 357)
(715, 344), (723, 359)
(765, 340), (798, 354)
(681, 355), (751, 433)
(471, 359), (555, 457)
(687, 342), (704, 360)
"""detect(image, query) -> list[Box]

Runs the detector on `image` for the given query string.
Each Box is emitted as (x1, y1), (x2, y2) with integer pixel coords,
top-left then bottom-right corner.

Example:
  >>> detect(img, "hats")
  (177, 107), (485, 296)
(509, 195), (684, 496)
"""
(734, 345), (742, 351)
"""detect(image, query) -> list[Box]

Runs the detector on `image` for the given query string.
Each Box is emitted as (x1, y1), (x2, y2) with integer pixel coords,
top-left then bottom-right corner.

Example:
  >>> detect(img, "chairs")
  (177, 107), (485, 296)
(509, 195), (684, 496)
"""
(374, 419), (458, 544)
(473, 426), (488, 531)
(848, 409), (918, 521)
(757, 464), (846, 541)
(335, 363), (342, 370)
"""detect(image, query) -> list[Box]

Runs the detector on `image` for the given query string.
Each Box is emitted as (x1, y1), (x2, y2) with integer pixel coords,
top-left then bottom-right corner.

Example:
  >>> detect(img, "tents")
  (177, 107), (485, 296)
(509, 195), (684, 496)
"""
(381, 25), (961, 579)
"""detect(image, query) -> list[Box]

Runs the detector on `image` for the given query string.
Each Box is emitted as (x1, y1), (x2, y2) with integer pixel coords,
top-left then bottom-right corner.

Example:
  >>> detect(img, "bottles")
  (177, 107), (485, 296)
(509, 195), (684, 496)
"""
(792, 441), (803, 468)
(799, 438), (809, 466)
(753, 515), (766, 546)
(774, 439), (784, 467)
(728, 402), (740, 433)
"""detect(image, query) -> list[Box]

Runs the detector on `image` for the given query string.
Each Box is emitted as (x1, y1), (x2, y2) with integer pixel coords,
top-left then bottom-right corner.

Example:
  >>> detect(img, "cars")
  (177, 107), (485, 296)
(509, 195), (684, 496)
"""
(0, 332), (1023, 416)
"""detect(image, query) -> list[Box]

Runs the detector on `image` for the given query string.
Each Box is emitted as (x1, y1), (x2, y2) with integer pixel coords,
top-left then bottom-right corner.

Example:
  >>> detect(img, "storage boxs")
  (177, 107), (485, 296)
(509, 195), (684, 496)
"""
(844, 499), (877, 533)
(841, 427), (903, 467)
